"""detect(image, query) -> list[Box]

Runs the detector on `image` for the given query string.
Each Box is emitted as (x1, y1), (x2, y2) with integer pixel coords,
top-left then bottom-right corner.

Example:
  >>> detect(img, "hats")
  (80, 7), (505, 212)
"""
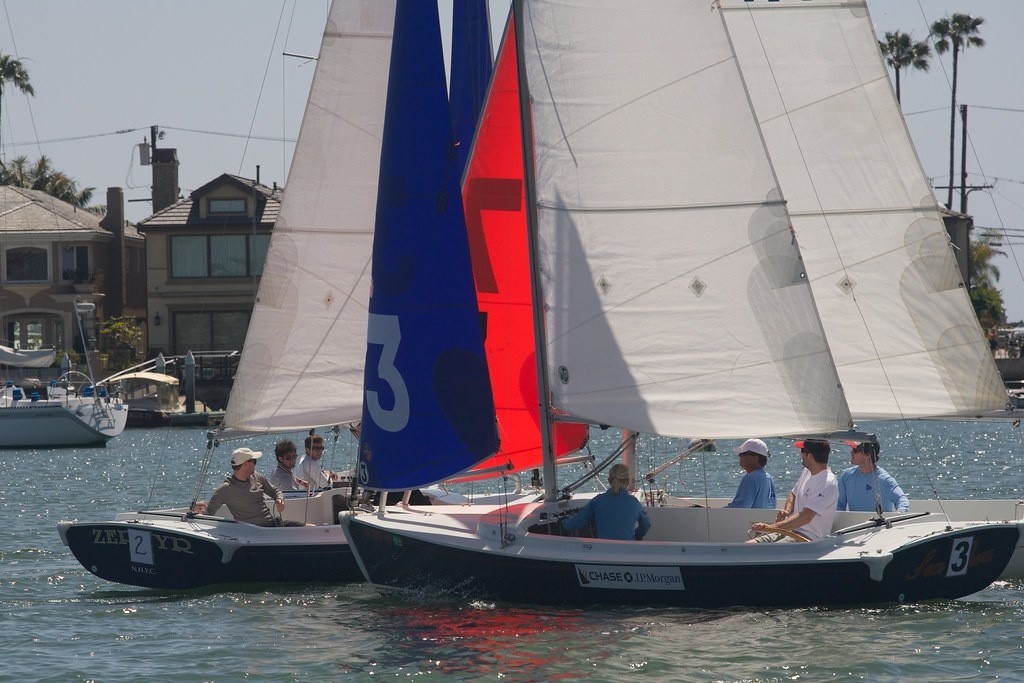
(846, 440), (879, 455)
(794, 438), (831, 457)
(231, 448), (262, 466)
(733, 438), (769, 457)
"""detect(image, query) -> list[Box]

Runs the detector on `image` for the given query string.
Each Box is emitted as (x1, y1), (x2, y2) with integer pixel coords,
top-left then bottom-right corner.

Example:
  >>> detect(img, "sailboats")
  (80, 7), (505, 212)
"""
(0, 0), (129, 449)
(339, 0), (1024, 608)
(56, 0), (542, 593)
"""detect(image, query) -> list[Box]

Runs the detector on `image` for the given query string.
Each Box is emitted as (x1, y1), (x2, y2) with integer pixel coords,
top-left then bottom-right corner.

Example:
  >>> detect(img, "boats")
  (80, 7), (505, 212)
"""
(110, 372), (184, 427)
(1008, 391), (1024, 410)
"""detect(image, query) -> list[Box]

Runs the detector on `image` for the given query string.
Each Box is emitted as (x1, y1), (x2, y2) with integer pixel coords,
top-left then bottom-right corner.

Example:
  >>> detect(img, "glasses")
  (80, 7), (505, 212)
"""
(282, 454), (297, 460)
(312, 446), (324, 451)
(250, 460), (257, 464)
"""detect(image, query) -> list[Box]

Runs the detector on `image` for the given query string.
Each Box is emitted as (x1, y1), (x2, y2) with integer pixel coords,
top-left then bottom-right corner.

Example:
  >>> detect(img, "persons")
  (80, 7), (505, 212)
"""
(988, 328), (998, 357)
(269, 440), (309, 492)
(1009, 332), (1024, 359)
(557, 464), (652, 541)
(193, 447), (316, 528)
(744, 439), (839, 544)
(837, 440), (909, 512)
(720, 438), (778, 510)
(358, 488), (431, 507)
(294, 434), (338, 491)
